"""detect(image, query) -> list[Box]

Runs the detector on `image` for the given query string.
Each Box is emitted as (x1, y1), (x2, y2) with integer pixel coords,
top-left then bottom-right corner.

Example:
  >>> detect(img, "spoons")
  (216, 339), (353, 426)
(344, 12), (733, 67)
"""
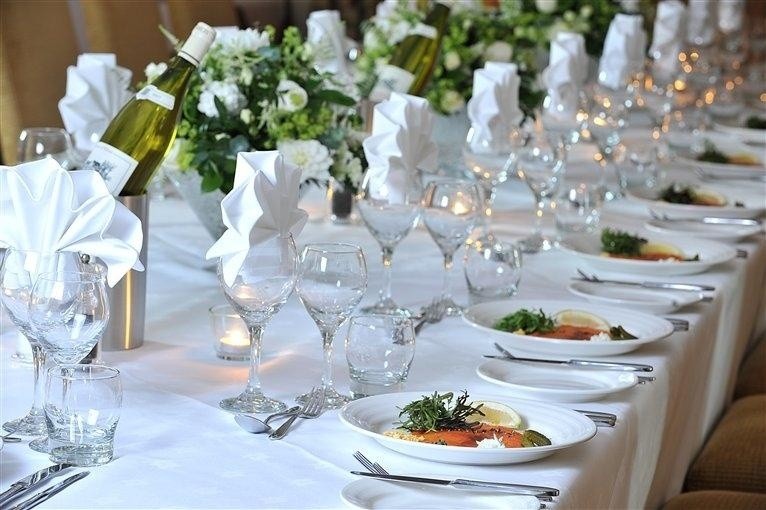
(233, 407), (301, 435)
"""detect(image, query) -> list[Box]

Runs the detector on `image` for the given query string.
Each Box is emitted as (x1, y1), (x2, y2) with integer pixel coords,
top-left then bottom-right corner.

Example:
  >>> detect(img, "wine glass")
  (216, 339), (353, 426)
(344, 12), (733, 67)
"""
(29, 271), (109, 452)
(295, 243), (367, 408)
(590, 95), (627, 199)
(217, 228), (298, 414)
(15, 127), (73, 169)
(424, 181), (477, 317)
(540, 89), (589, 182)
(357, 167), (424, 321)
(467, 127), (518, 223)
(0, 247), (85, 434)
(519, 132), (563, 255)
(627, 25), (766, 171)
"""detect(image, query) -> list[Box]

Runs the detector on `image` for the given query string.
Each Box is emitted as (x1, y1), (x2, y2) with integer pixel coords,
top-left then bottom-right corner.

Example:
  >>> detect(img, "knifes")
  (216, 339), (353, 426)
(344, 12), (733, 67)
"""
(481, 354), (652, 371)
(584, 278), (714, 292)
(0, 463), (73, 510)
(11, 471), (92, 510)
(350, 470), (559, 496)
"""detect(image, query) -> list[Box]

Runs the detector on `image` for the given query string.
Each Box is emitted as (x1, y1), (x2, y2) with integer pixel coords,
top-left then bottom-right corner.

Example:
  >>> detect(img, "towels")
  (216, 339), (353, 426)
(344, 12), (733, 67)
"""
(58, 51), (135, 153)
(362, 93), (441, 190)
(717, 0), (747, 33)
(686, 1), (716, 46)
(597, 13), (649, 89)
(465, 63), (525, 142)
(205, 150), (309, 287)
(649, 1), (687, 57)
(542, 33), (591, 95)
(0, 157), (144, 288)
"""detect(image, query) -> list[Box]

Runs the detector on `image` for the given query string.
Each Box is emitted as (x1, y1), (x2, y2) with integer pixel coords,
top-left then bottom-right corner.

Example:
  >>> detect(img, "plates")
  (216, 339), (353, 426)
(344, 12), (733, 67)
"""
(476, 357), (639, 401)
(566, 280), (703, 312)
(338, 392), (596, 464)
(624, 179), (766, 218)
(461, 299), (675, 356)
(674, 145), (766, 176)
(339, 476), (541, 509)
(646, 218), (763, 240)
(555, 223), (736, 274)
(710, 107), (766, 144)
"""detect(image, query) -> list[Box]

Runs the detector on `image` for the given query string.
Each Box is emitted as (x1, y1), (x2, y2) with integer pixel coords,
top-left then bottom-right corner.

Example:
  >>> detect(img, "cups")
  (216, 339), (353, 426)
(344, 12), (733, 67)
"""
(44, 364), (122, 466)
(346, 313), (417, 399)
(555, 174), (605, 232)
(207, 304), (264, 361)
(463, 239), (523, 298)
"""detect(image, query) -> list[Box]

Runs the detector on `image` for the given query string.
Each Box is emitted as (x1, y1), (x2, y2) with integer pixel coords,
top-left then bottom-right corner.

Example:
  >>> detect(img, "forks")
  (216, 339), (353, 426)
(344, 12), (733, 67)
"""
(414, 300), (445, 334)
(270, 386), (327, 441)
(494, 341), (655, 382)
(577, 268), (593, 281)
(352, 451), (547, 509)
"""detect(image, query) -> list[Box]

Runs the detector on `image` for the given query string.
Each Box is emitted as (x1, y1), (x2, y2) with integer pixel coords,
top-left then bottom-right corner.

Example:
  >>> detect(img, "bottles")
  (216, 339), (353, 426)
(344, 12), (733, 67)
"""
(82, 22), (216, 196)
(365, 0), (453, 102)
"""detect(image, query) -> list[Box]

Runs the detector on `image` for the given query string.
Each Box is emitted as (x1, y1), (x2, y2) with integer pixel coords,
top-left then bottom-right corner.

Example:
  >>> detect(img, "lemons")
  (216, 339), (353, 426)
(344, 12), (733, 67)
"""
(469, 400), (522, 427)
(555, 310), (611, 333)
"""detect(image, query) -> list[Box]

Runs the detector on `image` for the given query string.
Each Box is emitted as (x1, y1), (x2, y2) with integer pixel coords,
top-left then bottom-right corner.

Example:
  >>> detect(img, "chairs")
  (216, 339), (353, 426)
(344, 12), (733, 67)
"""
(1, 0), (80, 165)
(83, 0), (167, 90)
(661, 490), (766, 509)
(736, 336), (766, 396)
(684, 396), (765, 493)
(168, 0), (239, 47)
(239, 0), (288, 40)
(288, 0), (333, 35)
